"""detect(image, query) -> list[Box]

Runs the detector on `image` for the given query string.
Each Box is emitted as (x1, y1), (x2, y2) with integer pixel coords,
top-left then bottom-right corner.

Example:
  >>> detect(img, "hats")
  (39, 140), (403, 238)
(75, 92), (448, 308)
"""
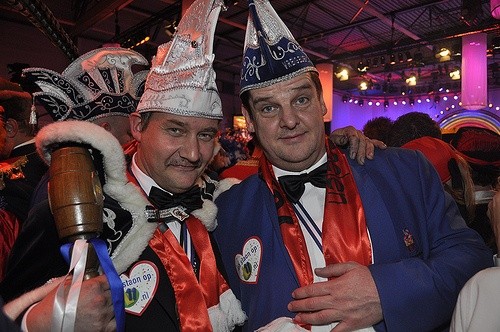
(136, 1), (228, 120)
(237, 0), (319, 96)
(18, 47), (151, 123)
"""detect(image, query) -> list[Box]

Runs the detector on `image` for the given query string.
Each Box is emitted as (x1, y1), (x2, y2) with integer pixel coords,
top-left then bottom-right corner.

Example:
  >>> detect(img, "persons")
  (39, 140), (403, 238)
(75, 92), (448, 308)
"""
(207, 0), (495, 332)
(0, 0), (388, 332)
(0, 48), (500, 332)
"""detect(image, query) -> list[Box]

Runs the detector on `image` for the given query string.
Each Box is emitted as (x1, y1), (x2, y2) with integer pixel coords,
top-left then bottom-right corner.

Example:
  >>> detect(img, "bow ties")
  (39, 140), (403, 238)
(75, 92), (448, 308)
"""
(278, 161), (329, 205)
(148, 184), (205, 210)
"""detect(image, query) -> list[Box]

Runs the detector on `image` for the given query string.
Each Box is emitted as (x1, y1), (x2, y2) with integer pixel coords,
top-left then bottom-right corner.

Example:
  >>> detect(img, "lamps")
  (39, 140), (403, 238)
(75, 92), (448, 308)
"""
(335, 42), (461, 108)
(120, 27), (150, 50)
